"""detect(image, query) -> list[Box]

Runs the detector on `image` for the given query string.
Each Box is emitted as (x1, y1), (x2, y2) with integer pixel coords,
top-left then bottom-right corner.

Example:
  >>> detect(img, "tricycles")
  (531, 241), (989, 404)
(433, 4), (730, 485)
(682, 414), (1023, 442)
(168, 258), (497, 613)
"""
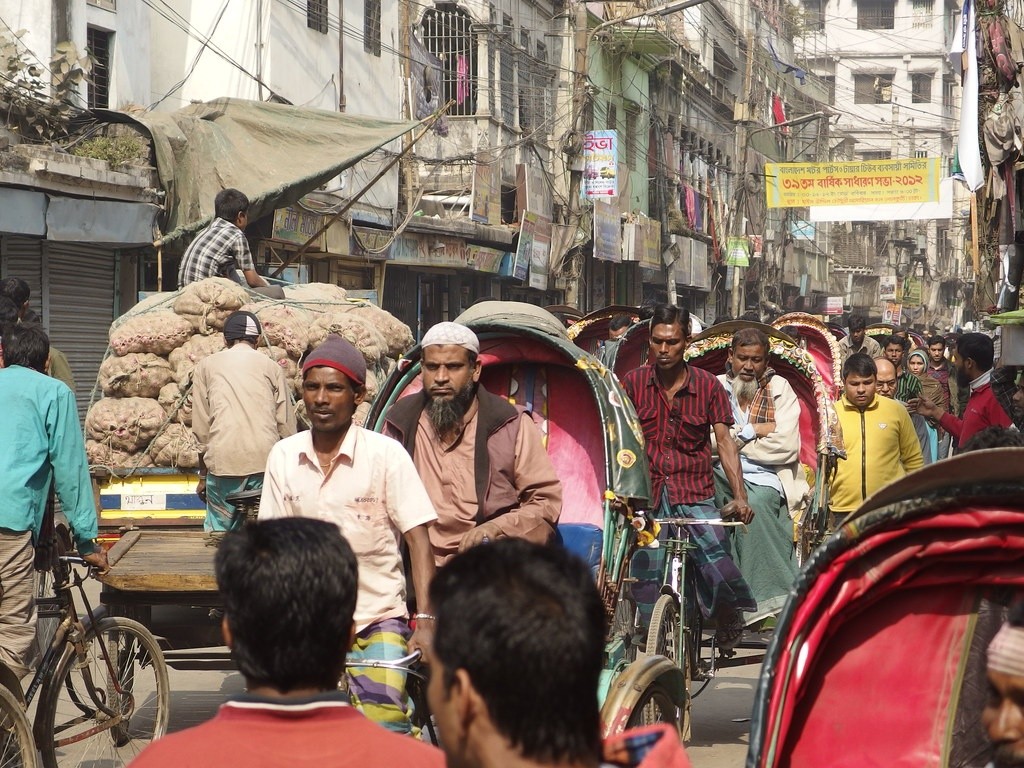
(565, 305), (925, 728)
(343, 301), (685, 730)
(0, 554), (170, 768)
(749, 447), (1023, 768)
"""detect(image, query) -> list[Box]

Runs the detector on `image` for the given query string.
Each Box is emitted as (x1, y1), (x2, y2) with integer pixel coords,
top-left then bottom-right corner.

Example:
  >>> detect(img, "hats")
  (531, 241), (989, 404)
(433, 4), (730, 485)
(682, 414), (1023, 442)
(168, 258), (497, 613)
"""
(223, 311), (262, 340)
(421, 322), (480, 355)
(302, 335), (366, 385)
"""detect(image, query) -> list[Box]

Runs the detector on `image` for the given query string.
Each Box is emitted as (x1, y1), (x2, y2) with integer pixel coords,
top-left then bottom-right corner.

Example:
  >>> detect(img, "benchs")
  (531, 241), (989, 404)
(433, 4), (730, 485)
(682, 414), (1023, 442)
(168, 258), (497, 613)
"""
(558, 521), (602, 581)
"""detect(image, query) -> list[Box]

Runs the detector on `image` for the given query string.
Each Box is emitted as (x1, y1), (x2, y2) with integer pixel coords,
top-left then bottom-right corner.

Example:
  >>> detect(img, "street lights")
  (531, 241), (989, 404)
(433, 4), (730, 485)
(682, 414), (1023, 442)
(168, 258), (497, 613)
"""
(721, 112), (825, 313)
(564, 1), (706, 322)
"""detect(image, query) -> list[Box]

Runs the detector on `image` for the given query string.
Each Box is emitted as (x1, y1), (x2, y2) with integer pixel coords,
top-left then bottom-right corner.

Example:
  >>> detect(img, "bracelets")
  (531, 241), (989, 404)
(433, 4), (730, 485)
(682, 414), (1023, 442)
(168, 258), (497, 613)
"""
(197, 471), (206, 480)
(415, 614), (436, 620)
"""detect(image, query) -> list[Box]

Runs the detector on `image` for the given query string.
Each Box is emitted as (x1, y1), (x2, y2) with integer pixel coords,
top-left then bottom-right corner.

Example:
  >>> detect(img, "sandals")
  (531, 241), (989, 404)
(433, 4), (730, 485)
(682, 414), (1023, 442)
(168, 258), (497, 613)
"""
(715, 609), (743, 651)
(631, 621), (665, 648)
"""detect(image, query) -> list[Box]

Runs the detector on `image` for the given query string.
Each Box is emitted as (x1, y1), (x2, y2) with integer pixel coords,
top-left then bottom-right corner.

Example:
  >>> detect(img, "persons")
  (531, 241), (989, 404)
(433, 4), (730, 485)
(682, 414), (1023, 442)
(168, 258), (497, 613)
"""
(873, 356), (898, 399)
(178, 190), (285, 303)
(887, 311), (891, 318)
(883, 334), (932, 467)
(0, 325), (111, 768)
(707, 328), (801, 634)
(893, 325), (908, 340)
(191, 310), (298, 534)
(426, 537), (692, 768)
(907, 346), (946, 463)
(840, 314), (882, 380)
(828, 353), (925, 527)
(620, 303), (755, 652)
(927, 335), (960, 460)
(609, 314), (636, 341)
(0, 276), (76, 393)
(126, 515), (448, 768)
(380, 321), (565, 617)
(981, 604), (1024, 768)
(255, 334), (438, 743)
(905, 333), (1024, 454)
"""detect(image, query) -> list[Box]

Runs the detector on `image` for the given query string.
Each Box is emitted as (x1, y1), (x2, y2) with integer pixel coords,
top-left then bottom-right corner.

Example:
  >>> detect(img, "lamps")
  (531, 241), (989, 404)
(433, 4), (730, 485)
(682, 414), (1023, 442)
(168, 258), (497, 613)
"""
(584, 0), (707, 73)
(744, 112), (824, 163)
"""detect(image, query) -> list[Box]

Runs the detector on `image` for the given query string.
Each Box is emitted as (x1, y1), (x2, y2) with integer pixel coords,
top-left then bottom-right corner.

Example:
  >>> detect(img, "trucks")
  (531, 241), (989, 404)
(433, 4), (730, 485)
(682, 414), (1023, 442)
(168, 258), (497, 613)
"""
(92, 467), (230, 631)
